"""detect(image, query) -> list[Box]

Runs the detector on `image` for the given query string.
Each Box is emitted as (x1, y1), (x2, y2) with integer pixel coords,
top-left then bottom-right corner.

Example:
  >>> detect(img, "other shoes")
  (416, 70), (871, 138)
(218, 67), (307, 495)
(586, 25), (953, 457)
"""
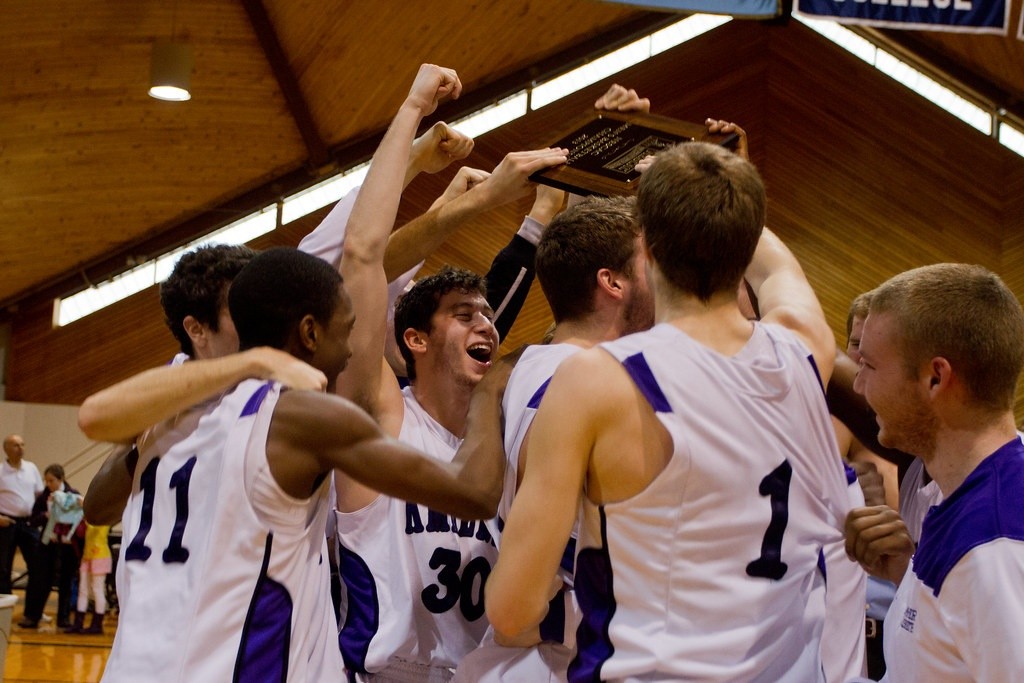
(39, 613), (53, 622)
(56, 621), (72, 628)
(17, 619), (37, 628)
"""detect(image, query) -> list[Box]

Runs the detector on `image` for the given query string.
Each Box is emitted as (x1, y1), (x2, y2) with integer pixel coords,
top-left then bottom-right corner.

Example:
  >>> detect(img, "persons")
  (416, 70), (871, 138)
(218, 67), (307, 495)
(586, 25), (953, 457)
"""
(77, 63), (1024, 683)
(0, 434), (111, 635)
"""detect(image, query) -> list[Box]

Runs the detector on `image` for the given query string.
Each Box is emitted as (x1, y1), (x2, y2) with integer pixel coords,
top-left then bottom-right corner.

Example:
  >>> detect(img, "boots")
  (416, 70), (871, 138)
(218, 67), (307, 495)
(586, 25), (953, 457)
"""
(63, 612), (104, 634)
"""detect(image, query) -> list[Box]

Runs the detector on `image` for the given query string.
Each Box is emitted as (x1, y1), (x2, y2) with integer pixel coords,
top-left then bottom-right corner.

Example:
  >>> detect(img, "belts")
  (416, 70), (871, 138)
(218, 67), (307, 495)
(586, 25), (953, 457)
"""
(866, 617), (884, 639)
